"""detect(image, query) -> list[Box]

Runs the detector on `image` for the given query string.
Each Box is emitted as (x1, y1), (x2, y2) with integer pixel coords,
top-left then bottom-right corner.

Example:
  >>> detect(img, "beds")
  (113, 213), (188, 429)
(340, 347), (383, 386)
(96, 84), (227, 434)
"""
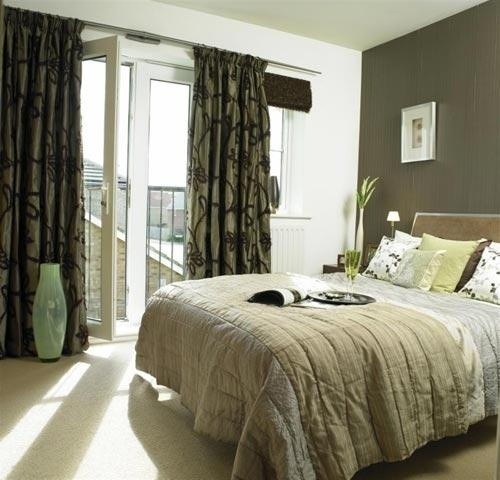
(138, 212), (500, 480)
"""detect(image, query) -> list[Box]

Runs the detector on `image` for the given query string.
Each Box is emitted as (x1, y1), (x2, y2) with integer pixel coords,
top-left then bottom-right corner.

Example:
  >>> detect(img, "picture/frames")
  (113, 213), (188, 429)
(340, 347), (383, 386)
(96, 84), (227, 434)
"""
(401, 100), (436, 164)
(364, 243), (379, 267)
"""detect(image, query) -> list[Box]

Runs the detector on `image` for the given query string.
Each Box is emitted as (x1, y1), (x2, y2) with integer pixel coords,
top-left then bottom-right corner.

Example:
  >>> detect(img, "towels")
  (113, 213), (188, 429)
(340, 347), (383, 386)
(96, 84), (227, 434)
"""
(245, 284), (310, 307)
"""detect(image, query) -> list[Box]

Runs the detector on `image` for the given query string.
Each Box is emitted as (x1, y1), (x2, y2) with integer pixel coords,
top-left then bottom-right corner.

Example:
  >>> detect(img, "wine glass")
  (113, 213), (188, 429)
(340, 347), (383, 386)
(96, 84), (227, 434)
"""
(345, 249), (362, 301)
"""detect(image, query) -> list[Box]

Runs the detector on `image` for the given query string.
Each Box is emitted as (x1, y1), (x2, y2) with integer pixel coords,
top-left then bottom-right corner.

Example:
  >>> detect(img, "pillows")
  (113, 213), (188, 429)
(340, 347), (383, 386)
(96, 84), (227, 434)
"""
(361, 229), (500, 307)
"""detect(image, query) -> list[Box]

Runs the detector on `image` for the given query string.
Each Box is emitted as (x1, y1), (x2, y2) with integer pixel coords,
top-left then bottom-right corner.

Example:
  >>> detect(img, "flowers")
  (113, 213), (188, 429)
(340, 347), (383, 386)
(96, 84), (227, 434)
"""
(352, 171), (381, 208)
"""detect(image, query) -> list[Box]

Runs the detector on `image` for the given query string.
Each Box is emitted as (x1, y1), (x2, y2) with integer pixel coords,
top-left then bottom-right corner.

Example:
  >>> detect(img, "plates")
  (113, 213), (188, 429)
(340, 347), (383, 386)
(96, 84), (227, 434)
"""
(306, 291), (376, 305)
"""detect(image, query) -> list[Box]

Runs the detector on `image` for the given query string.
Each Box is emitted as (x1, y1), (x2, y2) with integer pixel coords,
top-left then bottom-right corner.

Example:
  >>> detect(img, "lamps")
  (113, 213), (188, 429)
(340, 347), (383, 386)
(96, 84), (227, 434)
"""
(385, 209), (401, 241)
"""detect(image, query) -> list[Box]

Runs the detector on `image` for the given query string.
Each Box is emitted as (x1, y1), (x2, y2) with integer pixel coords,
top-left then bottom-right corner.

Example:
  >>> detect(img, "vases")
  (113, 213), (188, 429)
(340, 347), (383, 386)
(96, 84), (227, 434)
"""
(32, 259), (69, 362)
(356, 207), (365, 257)
